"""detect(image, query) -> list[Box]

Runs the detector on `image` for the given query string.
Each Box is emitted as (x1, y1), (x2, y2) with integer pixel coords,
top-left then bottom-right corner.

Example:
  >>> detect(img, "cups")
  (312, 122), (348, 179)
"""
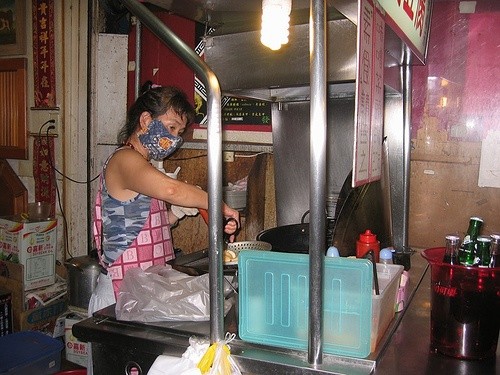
(27, 203), (50, 222)
(391, 245), (418, 271)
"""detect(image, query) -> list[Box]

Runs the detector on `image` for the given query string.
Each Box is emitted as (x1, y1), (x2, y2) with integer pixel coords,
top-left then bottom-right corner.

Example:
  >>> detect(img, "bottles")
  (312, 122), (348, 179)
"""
(471, 236), (492, 268)
(442, 235), (460, 265)
(488, 233), (500, 268)
(357, 229), (381, 262)
(379, 248), (395, 264)
(326, 246), (340, 257)
(458, 216), (484, 266)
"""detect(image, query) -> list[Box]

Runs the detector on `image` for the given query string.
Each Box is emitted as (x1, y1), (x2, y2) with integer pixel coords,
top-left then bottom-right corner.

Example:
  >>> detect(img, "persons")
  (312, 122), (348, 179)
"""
(87, 80), (240, 375)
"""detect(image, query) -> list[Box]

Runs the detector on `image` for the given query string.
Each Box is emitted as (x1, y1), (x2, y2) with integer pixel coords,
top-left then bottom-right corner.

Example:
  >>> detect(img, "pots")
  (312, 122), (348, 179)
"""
(63, 249), (99, 309)
(257, 210), (334, 254)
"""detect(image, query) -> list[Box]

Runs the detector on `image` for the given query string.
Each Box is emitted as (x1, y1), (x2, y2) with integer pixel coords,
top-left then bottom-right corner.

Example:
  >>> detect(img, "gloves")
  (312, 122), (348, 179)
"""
(171, 186), (202, 219)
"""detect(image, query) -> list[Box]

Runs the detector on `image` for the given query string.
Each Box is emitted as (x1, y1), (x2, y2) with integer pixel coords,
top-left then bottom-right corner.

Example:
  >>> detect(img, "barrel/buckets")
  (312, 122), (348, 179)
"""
(420, 246), (500, 362)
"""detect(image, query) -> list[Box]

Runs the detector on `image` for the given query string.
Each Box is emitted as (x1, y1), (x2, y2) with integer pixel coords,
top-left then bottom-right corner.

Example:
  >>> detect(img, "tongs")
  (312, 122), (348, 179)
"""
(360, 248), (381, 296)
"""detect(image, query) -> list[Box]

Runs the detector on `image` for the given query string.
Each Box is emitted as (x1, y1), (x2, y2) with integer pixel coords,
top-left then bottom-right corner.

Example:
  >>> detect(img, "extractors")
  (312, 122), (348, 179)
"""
(201, 0), (403, 106)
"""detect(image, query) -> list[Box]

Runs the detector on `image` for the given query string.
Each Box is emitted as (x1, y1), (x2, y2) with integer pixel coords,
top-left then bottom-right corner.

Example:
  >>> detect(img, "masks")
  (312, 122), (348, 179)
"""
(138, 120), (181, 160)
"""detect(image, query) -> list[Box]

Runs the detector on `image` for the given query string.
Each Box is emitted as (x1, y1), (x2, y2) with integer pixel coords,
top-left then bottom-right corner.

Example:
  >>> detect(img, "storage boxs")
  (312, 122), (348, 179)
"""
(0, 216), (95, 375)
(291, 263), (405, 354)
